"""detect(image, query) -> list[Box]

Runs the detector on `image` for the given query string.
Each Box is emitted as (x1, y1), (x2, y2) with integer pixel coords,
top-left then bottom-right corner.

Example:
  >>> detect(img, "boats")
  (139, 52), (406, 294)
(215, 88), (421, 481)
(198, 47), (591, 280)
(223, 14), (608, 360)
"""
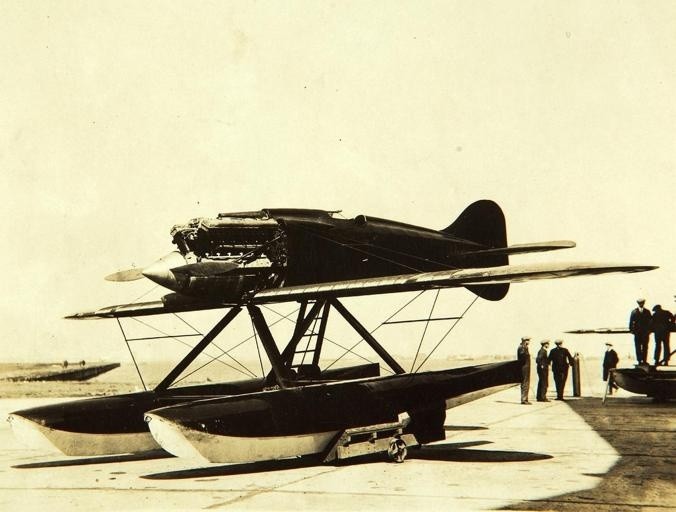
(0, 360), (122, 383)
(607, 359), (676, 403)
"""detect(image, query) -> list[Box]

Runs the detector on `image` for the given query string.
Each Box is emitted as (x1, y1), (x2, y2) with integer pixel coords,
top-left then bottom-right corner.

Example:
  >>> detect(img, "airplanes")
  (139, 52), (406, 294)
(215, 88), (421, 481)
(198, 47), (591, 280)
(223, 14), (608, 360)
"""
(5, 198), (658, 468)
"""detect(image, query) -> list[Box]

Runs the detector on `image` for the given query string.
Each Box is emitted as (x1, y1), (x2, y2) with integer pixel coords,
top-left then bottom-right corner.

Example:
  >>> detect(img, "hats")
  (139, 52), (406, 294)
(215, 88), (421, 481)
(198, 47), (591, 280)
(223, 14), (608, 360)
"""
(637, 297), (645, 303)
(521, 336), (563, 345)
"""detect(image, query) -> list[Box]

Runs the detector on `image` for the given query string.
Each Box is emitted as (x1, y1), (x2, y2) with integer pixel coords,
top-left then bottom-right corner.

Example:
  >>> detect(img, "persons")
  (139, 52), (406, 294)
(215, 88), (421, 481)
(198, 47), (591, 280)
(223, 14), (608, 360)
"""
(629, 297), (651, 365)
(545, 339), (574, 400)
(516, 337), (532, 405)
(651, 304), (674, 366)
(603, 343), (618, 394)
(536, 338), (551, 402)
